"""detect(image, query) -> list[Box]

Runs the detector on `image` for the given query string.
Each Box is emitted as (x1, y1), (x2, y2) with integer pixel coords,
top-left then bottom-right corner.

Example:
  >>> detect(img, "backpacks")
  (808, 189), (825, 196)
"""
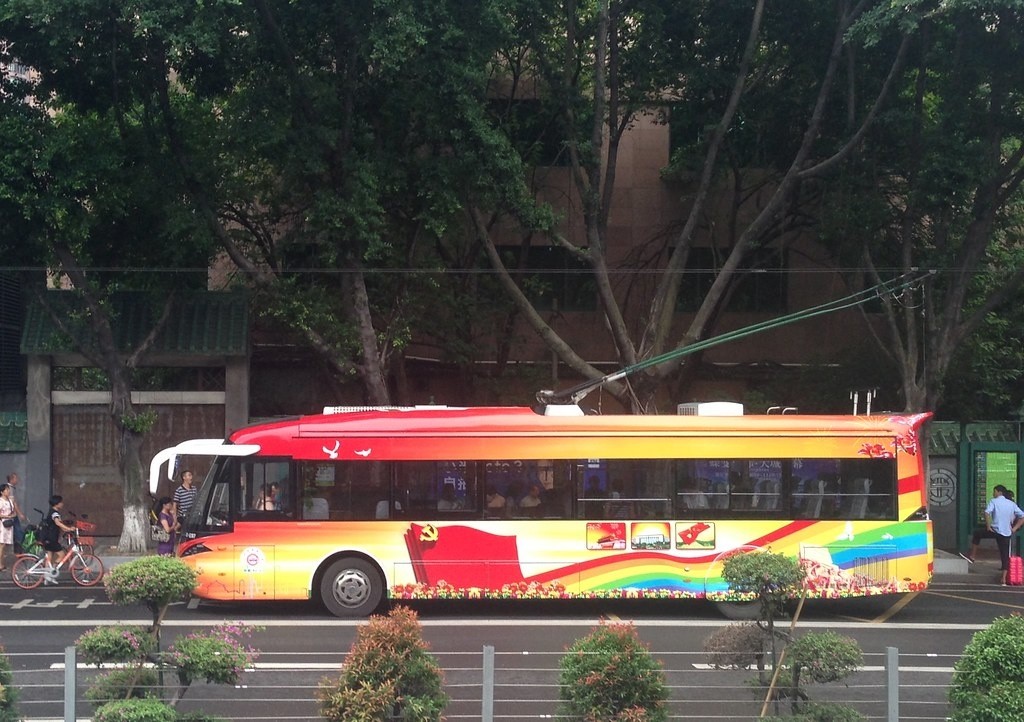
(35, 509), (60, 542)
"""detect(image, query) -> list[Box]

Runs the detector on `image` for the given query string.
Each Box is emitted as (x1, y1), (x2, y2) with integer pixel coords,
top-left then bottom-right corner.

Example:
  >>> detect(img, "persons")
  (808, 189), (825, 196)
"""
(0, 484), (16, 573)
(256, 482), (278, 510)
(486, 481), (571, 517)
(819, 471), (841, 514)
(42, 495), (75, 586)
(174, 471), (198, 527)
(586, 475), (628, 517)
(958, 485), (1024, 586)
(439, 484), (465, 510)
(7, 472), (27, 553)
(157, 497), (181, 555)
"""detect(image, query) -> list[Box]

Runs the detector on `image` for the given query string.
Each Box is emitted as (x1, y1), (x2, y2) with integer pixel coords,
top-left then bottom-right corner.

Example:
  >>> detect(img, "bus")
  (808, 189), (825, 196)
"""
(150, 401), (935, 621)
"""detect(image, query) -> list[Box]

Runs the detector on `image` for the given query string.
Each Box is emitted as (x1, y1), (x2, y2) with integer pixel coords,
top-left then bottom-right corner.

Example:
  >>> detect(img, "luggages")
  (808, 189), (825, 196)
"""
(1005, 537), (1024, 586)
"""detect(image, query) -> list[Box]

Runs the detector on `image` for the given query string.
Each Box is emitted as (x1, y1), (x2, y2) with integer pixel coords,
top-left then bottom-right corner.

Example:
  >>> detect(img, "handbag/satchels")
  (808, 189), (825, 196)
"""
(151, 525), (170, 542)
(3, 519), (12, 527)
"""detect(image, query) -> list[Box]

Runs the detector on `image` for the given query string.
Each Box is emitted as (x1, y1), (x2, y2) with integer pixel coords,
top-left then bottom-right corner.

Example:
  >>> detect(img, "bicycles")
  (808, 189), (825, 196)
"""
(147, 490), (159, 521)
(11, 508), (104, 589)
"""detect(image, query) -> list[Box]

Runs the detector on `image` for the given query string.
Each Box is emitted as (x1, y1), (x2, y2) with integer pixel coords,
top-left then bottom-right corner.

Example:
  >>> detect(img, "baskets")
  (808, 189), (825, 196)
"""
(75, 521), (97, 545)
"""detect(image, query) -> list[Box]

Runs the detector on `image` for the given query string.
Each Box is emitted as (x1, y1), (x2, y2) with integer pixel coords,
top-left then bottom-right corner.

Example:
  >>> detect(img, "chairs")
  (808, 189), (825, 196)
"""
(374, 500), (402, 519)
(709, 481), (729, 509)
(800, 479), (827, 517)
(848, 479), (872, 516)
(302, 497), (329, 520)
(752, 479), (781, 510)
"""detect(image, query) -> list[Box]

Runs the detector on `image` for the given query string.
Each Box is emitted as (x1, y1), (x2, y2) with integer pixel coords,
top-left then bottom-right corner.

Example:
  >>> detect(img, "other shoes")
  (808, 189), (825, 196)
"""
(44, 580), (55, 585)
(999, 579), (1006, 586)
(47, 577), (59, 584)
(959, 552), (974, 564)
(0, 567), (7, 571)
